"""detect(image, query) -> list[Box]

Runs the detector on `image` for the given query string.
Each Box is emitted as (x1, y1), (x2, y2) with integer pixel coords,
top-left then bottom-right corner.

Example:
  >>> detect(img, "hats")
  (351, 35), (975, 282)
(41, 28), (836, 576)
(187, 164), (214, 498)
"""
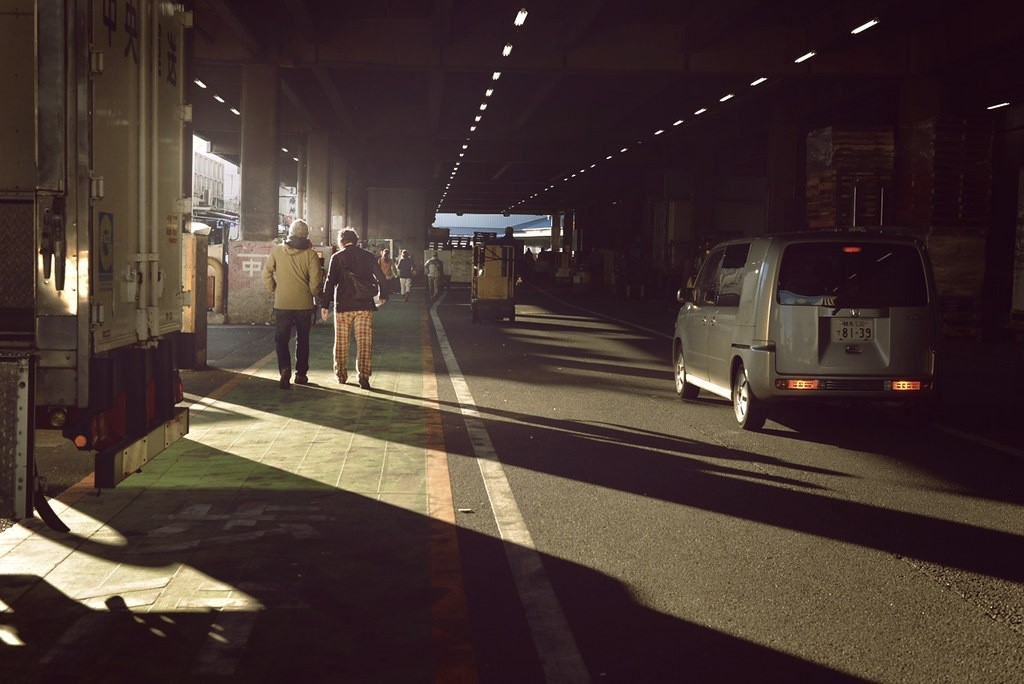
(431, 252), (438, 255)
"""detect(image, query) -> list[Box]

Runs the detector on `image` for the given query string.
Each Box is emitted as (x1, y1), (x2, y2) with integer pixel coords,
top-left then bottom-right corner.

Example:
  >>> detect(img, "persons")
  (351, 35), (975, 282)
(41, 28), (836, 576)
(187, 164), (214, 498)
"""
(320, 229), (390, 389)
(377, 248), (444, 303)
(264, 220), (321, 389)
(524, 248), (547, 275)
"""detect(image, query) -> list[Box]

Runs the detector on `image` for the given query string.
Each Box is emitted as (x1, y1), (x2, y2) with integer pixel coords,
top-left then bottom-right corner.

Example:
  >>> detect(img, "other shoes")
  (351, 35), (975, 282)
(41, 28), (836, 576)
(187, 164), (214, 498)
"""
(339, 380), (346, 384)
(279, 378), (290, 389)
(405, 295), (408, 302)
(295, 373), (308, 383)
(359, 378), (370, 390)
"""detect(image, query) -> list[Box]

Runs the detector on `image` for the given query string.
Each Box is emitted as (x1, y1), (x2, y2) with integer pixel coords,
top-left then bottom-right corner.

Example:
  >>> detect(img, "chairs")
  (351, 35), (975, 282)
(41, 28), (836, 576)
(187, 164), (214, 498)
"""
(809, 255), (836, 294)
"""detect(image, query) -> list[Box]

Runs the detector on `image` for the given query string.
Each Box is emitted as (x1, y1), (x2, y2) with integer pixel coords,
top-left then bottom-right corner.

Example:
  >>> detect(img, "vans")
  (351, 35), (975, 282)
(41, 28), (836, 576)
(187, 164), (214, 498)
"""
(670, 226), (943, 431)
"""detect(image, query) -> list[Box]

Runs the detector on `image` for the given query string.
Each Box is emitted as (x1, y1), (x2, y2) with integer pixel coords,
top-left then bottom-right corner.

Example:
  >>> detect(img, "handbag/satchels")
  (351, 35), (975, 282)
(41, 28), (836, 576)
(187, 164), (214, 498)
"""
(391, 277), (400, 290)
(338, 271), (375, 306)
(412, 269), (416, 276)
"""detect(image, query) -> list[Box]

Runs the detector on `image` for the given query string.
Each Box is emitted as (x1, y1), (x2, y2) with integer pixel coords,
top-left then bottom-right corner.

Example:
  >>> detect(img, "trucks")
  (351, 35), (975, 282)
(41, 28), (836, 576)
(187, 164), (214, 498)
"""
(0, 0), (192, 490)
